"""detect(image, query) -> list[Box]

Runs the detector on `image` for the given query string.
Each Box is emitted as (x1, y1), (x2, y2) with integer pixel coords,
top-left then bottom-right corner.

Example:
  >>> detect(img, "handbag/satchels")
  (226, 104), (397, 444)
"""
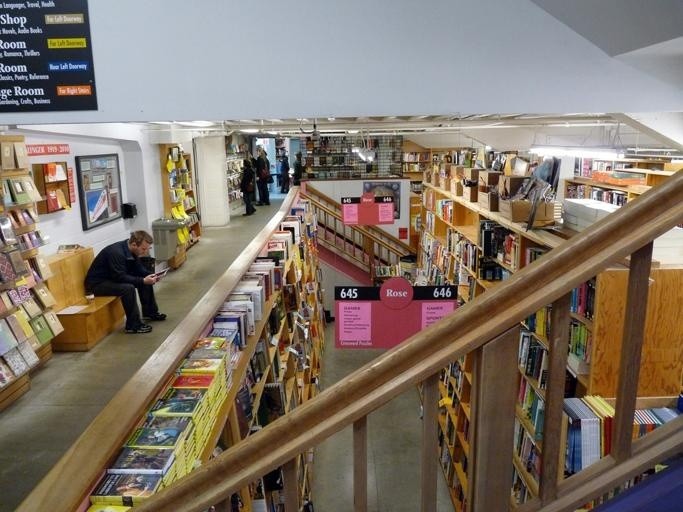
(260, 167), (269, 178)
(246, 183), (254, 193)
(267, 175), (274, 183)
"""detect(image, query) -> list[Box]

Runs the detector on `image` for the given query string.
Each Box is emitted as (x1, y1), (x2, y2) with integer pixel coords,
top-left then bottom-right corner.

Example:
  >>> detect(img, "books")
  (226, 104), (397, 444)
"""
(56, 244), (87, 256)
(224, 143), (249, 203)
(403, 151), (430, 171)
(592, 161), (634, 172)
(0, 140), (67, 391)
(227, 194), (328, 300)
(150, 265), (172, 279)
(371, 262), (410, 289)
(164, 143), (201, 253)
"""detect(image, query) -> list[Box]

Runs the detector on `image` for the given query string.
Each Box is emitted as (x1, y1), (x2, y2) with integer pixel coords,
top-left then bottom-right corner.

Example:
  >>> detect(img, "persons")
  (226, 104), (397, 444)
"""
(83, 231), (167, 334)
(292, 151), (306, 186)
(256, 148), (271, 205)
(239, 159), (257, 216)
(278, 154), (290, 193)
(248, 144), (269, 203)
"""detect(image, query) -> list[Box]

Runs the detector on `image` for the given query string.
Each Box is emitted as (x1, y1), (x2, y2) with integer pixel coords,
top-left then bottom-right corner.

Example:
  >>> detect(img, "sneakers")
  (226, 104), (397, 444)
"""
(255, 201), (270, 206)
(141, 312), (167, 321)
(242, 209), (256, 216)
(281, 190), (289, 193)
(123, 324), (153, 333)
